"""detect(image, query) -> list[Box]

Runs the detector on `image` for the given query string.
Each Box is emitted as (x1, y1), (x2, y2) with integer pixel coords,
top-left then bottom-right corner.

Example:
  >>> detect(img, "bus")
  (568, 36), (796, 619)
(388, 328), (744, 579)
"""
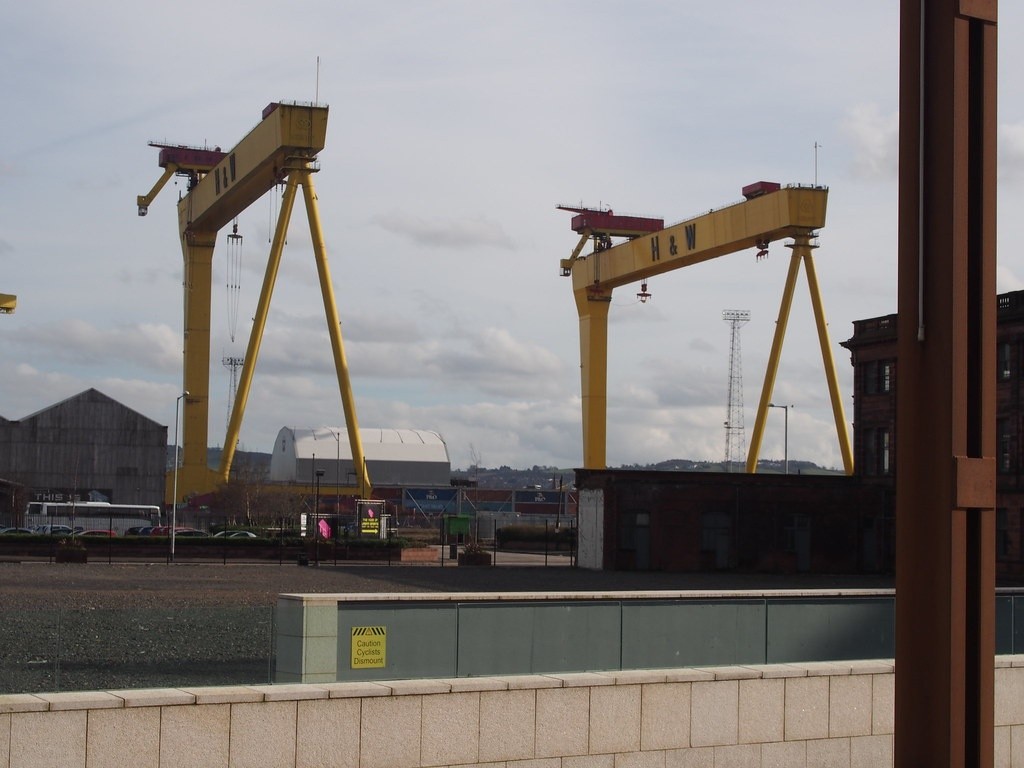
(25, 499), (162, 536)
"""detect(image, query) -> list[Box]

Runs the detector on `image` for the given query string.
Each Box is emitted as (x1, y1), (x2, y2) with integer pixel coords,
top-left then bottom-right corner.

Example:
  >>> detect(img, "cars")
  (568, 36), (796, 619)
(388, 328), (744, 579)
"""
(0, 522), (259, 538)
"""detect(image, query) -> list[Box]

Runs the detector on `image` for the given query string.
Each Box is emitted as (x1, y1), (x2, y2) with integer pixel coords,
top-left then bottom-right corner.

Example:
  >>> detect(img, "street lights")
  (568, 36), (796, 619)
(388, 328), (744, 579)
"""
(768, 403), (789, 475)
(169, 390), (191, 561)
(313, 468), (325, 566)
